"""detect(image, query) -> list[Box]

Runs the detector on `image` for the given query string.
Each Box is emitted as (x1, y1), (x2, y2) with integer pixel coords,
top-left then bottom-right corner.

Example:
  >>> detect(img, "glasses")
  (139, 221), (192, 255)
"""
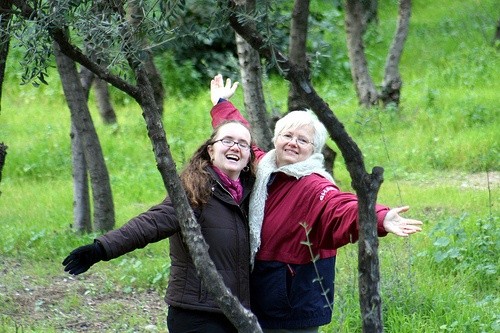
(278, 130), (314, 145)
(211, 138), (251, 151)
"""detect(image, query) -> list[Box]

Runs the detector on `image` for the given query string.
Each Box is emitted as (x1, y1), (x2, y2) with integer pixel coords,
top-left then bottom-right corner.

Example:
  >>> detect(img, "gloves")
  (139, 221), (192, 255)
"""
(62, 242), (105, 276)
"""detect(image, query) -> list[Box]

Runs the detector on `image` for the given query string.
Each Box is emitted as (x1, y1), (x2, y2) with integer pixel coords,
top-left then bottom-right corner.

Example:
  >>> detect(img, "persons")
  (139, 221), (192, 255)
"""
(61, 120), (258, 333)
(211, 73), (424, 333)
